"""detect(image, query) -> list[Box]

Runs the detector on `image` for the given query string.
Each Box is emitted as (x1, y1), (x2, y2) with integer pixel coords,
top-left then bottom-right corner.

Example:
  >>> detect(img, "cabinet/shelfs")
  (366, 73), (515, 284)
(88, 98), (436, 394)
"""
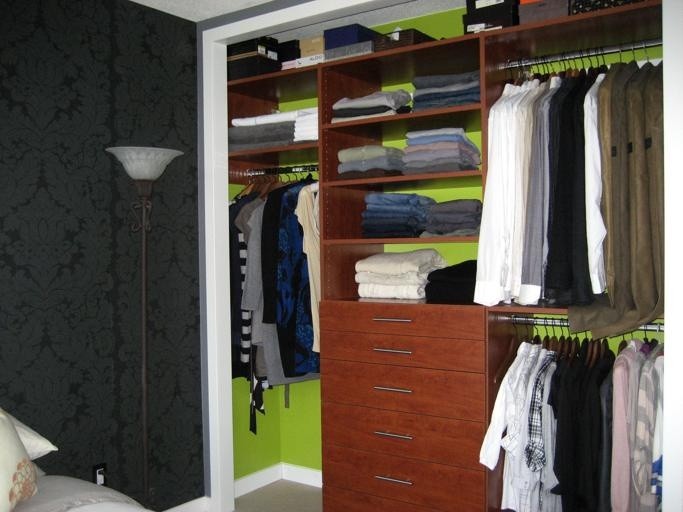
(227, 0), (682, 512)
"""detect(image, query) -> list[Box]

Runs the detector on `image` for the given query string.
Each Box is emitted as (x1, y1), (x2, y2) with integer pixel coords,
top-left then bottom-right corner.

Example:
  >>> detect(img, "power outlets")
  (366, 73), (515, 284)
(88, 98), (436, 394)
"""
(92, 463), (106, 486)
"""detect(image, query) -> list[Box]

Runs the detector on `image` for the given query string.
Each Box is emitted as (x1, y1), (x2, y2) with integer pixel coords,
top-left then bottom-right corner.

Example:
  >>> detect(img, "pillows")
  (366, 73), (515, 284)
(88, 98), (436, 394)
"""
(0, 409), (62, 512)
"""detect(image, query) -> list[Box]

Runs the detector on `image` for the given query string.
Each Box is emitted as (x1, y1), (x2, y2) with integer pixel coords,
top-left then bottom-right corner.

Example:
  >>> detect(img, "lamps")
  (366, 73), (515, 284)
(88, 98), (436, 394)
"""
(106, 146), (184, 508)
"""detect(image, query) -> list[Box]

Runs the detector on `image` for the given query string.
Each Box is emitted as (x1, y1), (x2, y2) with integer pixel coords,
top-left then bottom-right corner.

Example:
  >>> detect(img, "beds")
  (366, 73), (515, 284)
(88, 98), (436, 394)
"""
(14, 473), (156, 512)
